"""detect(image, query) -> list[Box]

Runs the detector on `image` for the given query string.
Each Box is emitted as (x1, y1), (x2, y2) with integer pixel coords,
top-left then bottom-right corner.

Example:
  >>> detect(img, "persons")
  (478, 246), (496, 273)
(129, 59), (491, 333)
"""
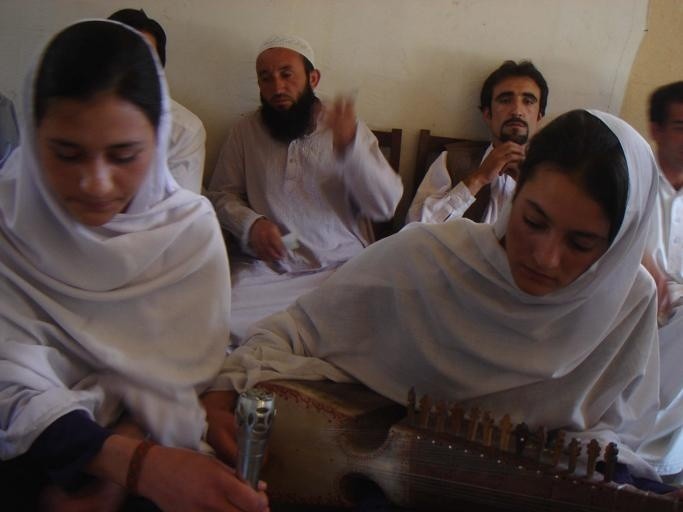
(199, 104), (664, 475)
(405, 61), (559, 229)
(199, 34), (409, 345)
(104, 7), (209, 191)
(0, 20), (273, 512)
(621, 81), (681, 475)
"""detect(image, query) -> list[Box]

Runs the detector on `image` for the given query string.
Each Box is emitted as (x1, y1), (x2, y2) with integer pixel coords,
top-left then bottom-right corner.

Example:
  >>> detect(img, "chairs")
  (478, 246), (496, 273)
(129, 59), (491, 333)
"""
(408, 127), (494, 225)
(368, 130), (401, 244)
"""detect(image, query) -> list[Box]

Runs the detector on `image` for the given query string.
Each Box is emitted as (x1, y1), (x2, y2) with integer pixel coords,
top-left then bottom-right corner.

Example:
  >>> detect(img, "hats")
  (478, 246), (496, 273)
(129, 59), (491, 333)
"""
(256, 30), (315, 68)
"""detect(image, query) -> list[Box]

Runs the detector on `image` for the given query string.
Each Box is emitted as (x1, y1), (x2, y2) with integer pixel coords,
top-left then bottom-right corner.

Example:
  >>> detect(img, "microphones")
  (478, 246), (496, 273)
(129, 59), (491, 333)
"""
(234, 388), (278, 494)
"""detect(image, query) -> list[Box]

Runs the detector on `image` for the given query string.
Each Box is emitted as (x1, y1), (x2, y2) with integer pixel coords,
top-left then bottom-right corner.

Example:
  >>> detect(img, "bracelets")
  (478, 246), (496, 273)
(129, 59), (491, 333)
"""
(123, 436), (151, 504)
